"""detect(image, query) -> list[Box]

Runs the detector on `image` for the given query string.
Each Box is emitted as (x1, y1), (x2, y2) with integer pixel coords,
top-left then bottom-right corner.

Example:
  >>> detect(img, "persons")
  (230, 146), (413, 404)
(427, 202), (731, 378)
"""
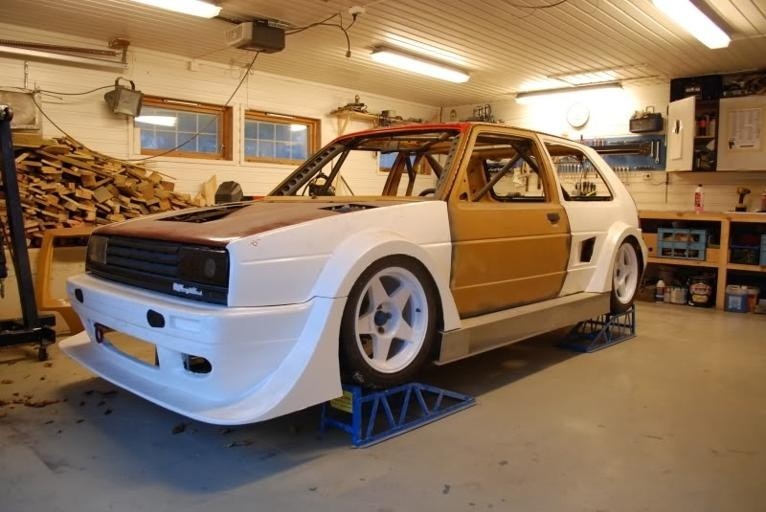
(481, 103), (497, 123)
(469, 105), (484, 122)
(449, 109), (457, 121)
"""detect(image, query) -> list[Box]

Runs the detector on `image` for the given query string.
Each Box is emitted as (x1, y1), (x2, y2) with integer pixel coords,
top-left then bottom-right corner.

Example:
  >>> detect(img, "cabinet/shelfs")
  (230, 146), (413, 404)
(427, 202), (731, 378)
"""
(633, 208), (765, 318)
(663, 72), (766, 175)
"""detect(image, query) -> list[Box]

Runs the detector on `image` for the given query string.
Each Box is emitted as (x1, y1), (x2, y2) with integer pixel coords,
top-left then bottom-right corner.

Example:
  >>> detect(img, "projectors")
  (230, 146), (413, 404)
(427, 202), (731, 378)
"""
(225, 22), (285, 54)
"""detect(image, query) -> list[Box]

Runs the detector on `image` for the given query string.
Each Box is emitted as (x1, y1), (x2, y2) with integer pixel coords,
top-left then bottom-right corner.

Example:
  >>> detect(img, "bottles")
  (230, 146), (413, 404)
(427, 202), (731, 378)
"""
(656, 278), (666, 304)
(699, 111), (707, 136)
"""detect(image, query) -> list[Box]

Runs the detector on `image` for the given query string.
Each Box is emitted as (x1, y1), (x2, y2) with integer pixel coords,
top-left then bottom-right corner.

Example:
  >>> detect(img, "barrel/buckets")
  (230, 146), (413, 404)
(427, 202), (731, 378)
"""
(687, 275), (716, 309)
(746, 289), (760, 312)
(724, 284), (747, 312)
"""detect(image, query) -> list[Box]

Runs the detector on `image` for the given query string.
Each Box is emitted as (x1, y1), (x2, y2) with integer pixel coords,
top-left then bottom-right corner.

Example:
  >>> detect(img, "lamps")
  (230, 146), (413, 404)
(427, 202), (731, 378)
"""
(369, 46), (471, 85)
(102, 75), (146, 117)
(515, 81), (624, 107)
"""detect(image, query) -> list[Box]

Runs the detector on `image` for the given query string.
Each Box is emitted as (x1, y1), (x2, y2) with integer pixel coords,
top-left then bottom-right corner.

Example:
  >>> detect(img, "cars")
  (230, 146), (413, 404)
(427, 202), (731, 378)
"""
(55, 118), (654, 433)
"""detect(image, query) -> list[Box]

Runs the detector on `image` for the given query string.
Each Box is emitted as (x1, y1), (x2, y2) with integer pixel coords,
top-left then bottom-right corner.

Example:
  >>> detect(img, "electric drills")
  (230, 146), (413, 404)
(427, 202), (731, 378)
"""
(735, 186), (751, 212)
